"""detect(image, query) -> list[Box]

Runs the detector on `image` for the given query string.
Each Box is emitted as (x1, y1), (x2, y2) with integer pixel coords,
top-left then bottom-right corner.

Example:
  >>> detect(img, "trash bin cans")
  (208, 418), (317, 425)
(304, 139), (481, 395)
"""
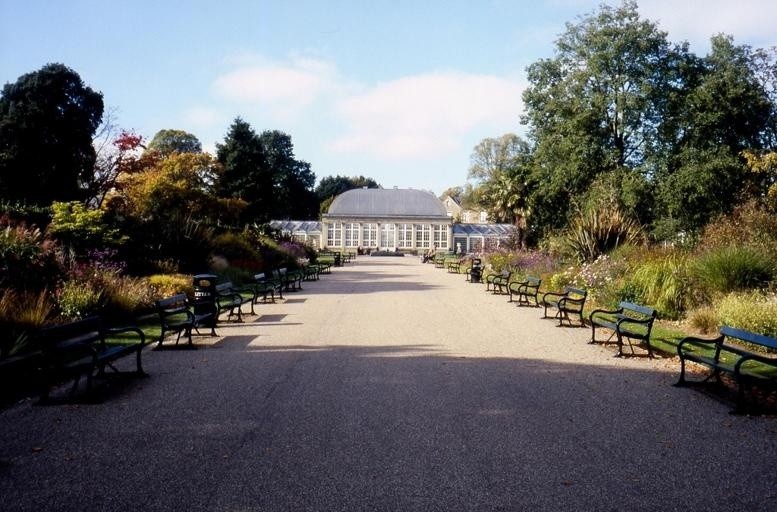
(335, 254), (340, 268)
(192, 274), (218, 328)
(471, 258), (482, 283)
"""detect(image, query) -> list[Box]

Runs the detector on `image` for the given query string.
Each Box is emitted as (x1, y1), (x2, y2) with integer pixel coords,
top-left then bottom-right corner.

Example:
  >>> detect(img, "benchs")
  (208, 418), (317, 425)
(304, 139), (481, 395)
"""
(41, 315), (148, 404)
(672, 326), (777, 414)
(155, 293), (218, 350)
(216, 286), (257, 324)
(508, 277), (542, 308)
(251, 252), (355, 304)
(422, 250), (485, 284)
(588, 301), (658, 358)
(485, 270), (512, 295)
(542, 286), (588, 327)
